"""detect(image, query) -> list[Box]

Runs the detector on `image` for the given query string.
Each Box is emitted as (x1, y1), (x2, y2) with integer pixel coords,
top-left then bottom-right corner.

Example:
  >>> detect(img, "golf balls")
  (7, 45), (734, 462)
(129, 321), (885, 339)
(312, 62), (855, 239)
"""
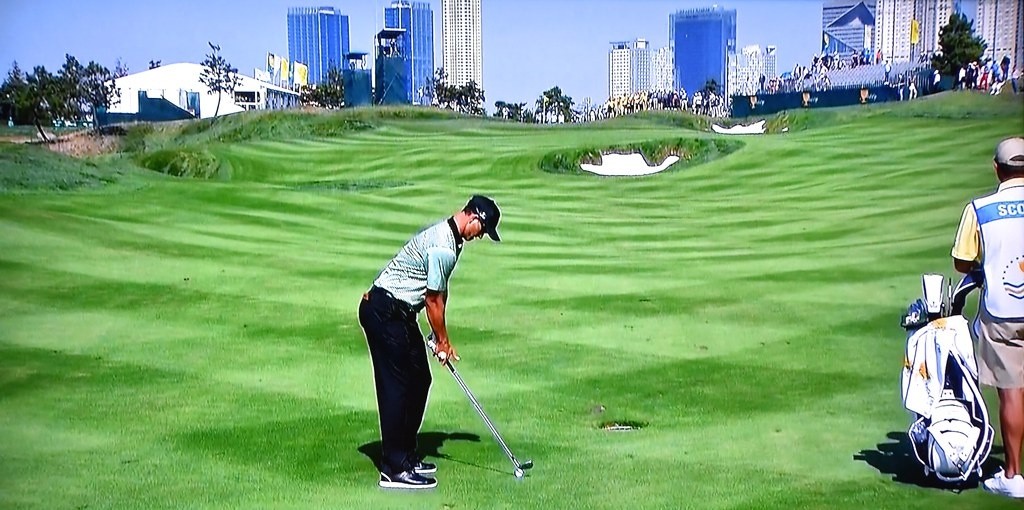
(514, 468), (524, 478)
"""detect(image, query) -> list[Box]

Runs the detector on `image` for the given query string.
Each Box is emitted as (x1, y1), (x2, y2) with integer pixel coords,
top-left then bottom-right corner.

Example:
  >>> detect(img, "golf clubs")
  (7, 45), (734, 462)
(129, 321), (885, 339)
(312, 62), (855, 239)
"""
(427, 334), (534, 469)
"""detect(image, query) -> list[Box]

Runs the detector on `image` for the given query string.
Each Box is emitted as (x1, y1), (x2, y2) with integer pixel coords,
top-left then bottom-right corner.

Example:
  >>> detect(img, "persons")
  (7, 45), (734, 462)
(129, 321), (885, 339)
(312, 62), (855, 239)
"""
(759, 51), (846, 95)
(692, 79), (726, 117)
(502, 106), (509, 119)
(951, 136), (1023, 499)
(850, 49), (882, 69)
(954, 54), (1020, 96)
(551, 87), (688, 124)
(883, 60), (918, 101)
(933, 70), (940, 91)
(359, 195), (500, 488)
(917, 47), (943, 72)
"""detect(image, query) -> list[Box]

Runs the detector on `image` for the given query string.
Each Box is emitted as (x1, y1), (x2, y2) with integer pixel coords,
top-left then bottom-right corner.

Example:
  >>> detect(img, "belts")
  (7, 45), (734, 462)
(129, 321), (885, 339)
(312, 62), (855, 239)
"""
(371, 285), (416, 321)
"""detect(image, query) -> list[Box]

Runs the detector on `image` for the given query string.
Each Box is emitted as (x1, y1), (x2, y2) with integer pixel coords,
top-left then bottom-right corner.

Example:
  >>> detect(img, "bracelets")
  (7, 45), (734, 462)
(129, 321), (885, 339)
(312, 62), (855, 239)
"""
(438, 341), (446, 344)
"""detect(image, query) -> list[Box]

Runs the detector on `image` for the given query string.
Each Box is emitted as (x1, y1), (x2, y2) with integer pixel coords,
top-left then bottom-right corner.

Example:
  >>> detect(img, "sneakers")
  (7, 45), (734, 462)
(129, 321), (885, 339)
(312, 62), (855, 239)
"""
(378, 465), (438, 489)
(412, 460), (437, 473)
(984, 466), (1024, 498)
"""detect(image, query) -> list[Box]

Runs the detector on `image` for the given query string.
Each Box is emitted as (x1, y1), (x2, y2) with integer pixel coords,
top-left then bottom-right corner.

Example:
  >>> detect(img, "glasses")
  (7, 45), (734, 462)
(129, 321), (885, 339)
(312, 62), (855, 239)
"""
(474, 212), (488, 234)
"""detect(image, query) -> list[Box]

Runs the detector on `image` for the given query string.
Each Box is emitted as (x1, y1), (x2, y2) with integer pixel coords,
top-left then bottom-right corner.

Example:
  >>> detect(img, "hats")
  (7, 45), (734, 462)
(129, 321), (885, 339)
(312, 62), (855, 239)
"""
(994, 137), (1024, 166)
(472, 195), (501, 242)
